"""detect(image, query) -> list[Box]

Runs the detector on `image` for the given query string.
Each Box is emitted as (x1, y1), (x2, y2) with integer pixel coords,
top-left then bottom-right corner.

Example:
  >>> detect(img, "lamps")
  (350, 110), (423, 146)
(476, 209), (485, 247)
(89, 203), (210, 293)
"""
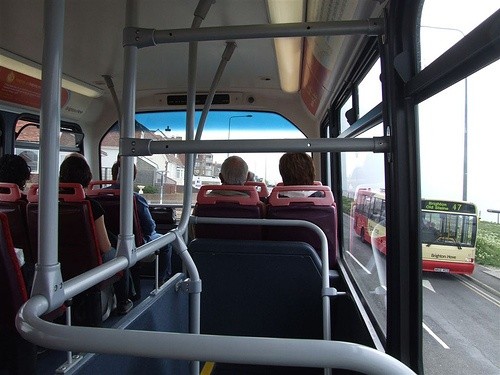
(0, 53), (104, 102)
(265, 0), (306, 96)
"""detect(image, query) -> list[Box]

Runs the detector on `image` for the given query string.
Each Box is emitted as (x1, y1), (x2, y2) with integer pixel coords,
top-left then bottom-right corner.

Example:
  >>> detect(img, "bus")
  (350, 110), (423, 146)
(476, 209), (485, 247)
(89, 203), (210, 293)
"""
(350, 185), (478, 273)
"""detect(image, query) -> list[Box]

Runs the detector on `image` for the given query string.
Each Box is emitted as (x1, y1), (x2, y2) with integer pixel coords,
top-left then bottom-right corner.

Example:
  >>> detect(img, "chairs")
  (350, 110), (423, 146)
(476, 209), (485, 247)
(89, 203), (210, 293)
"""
(0, 212), (67, 323)
(0, 182), (26, 252)
(244, 182), (267, 198)
(85, 180), (157, 263)
(264, 186), (339, 268)
(25, 182), (123, 290)
(192, 185), (265, 240)
(277, 181), (322, 186)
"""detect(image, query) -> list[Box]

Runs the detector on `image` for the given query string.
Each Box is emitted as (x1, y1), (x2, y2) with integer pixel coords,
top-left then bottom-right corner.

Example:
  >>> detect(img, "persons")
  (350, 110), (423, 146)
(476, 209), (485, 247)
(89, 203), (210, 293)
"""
(188, 156), (267, 239)
(100, 160), (173, 285)
(266, 153), (331, 198)
(59, 154), (133, 315)
(1, 153), (32, 201)
(12, 240), (80, 355)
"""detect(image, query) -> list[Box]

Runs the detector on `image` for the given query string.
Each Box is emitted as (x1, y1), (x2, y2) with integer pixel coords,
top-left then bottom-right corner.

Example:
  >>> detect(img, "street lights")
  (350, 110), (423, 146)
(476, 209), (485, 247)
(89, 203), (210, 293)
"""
(227, 114), (253, 158)
